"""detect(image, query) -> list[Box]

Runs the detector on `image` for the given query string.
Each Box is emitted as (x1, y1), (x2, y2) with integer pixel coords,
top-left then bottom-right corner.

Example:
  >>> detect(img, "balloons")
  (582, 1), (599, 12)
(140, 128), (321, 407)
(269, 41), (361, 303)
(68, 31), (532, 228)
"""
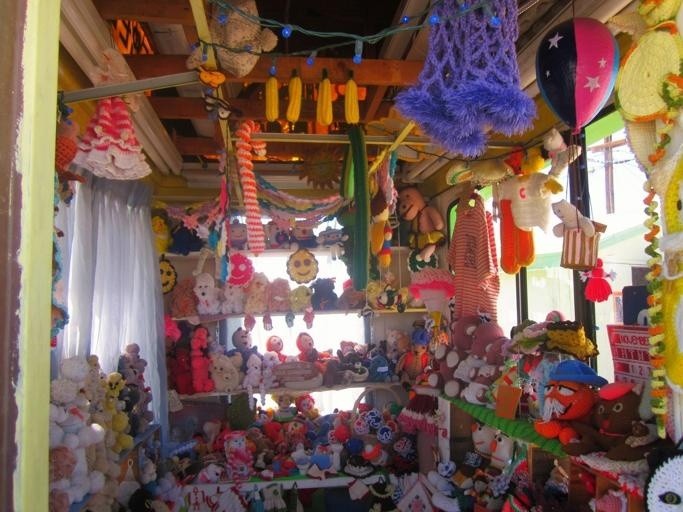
(537, 16), (619, 136)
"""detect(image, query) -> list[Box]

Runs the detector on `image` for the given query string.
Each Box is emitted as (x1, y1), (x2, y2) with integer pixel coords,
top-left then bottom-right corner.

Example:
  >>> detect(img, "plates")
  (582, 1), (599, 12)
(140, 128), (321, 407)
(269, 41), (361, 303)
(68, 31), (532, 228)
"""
(164, 244), (428, 501)
(410, 385), (652, 512)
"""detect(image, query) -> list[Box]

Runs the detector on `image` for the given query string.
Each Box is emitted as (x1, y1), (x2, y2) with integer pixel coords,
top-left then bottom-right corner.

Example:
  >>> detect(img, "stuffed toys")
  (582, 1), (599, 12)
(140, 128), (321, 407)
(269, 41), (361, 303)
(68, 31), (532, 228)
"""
(296, 333), (329, 375)
(187, 1), (277, 77)
(501, 461), (648, 512)
(55, 119), (79, 172)
(52, 343), (182, 510)
(228, 218), (325, 252)
(316, 68), (333, 125)
(329, 403), (477, 510)
(168, 393), (339, 484)
(74, 48), (152, 180)
(345, 69), (360, 124)
(149, 147), (229, 256)
(287, 247), (319, 284)
(286, 69), (302, 123)
(159, 254), (178, 294)
(171, 271), (426, 332)
(166, 314), (296, 409)
(51, 170), (75, 345)
(371, 192), (393, 267)
(228, 253), (254, 287)
(644, 437), (682, 511)
(563, 383), (652, 461)
(450, 418), (518, 500)
(192, 231), (221, 278)
(553, 199), (595, 239)
(266, 64), (279, 122)
(318, 226), (349, 259)
(338, 310), (609, 446)
(398, 187), (444, 262)
